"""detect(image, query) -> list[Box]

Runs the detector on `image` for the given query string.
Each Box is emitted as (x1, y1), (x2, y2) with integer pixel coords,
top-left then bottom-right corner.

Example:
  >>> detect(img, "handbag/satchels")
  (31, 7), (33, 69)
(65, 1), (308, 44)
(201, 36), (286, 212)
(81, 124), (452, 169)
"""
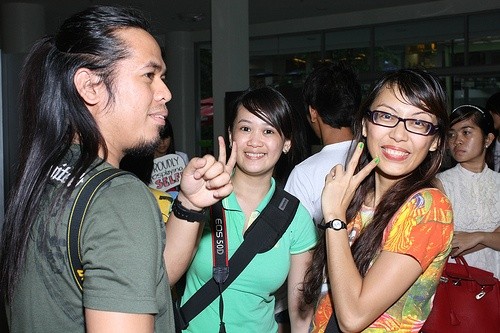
(419, 251), (500, 333)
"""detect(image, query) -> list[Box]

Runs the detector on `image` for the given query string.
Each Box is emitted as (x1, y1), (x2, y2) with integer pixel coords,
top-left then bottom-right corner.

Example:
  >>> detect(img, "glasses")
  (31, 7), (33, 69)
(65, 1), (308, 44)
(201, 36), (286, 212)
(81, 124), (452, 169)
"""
(368, 109), (440, 136)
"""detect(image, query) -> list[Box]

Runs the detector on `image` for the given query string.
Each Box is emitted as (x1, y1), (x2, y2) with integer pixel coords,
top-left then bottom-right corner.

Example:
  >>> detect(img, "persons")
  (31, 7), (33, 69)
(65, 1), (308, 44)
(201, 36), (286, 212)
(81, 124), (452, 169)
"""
(485, 92), (500, 173)
(283, 70), (368, 229)
(0, 6), (232, 333)
(176, 85), (321, 333)
(135, 116), (190, 191)
(430, 103), (500, 284)
(295, 65), (455, 333)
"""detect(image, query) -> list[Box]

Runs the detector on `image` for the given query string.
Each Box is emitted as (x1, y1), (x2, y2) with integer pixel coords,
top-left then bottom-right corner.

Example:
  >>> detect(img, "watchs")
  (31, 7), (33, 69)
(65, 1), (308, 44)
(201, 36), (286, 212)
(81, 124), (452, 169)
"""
(317, 219), (348, 230)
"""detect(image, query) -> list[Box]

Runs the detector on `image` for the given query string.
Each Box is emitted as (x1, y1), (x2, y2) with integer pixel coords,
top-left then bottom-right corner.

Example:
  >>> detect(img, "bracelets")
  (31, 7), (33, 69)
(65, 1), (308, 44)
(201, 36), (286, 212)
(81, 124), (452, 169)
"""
(170, 194), (212, 223)
